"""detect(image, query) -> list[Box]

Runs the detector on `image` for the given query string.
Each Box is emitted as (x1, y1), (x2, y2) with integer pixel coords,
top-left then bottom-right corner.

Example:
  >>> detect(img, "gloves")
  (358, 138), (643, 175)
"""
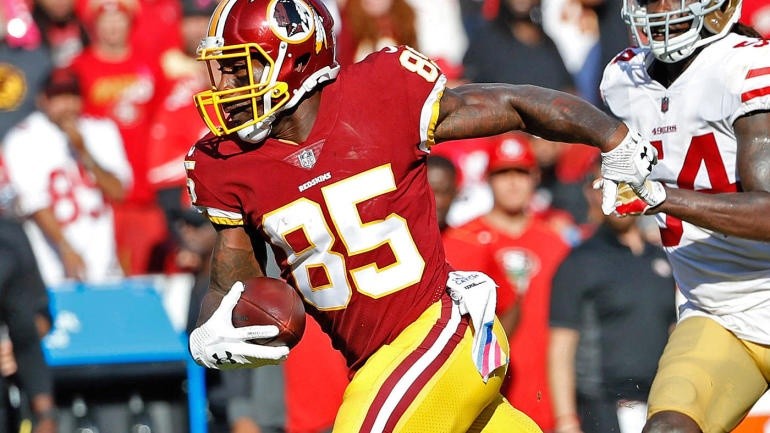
(593, 126), (658, 214)
(187, 275), (293, 373)
(588, 177), (667, 216)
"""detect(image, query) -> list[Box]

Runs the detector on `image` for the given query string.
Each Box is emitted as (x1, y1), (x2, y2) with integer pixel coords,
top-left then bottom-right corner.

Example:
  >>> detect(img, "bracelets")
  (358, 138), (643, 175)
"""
(34, 407), (57, 425)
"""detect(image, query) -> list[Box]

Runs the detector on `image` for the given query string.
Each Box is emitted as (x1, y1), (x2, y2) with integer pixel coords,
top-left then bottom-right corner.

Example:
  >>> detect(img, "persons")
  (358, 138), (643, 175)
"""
(442, 125), (586, 433)
(0, 241), (60, 433)
(0, 0), (769, 308)
(0, 212), (54, 376)
(594, 0), (770, 433)
(220, 362), (289, 433)
(172, 207), (231, 433)
(184, 0), (659, 433)
(543, 147), (677, 433)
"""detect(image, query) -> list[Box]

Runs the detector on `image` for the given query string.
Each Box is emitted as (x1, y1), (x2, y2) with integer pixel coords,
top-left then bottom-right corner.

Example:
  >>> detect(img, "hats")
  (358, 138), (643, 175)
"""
(167, 185), (215, 226)
(41, 67), (79, 98)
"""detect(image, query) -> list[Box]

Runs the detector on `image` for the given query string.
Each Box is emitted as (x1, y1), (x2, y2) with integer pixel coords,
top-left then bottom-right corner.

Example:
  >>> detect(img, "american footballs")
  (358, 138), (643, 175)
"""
(230, 276), (308, 354)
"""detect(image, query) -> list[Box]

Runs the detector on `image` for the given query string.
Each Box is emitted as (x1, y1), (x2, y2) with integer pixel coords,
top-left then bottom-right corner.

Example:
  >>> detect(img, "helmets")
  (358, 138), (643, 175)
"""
(481, 135), (543, 176)
(73, 0), (141, 31)
(622, 0), (739, 64)
(194, 0), (339, 145)
